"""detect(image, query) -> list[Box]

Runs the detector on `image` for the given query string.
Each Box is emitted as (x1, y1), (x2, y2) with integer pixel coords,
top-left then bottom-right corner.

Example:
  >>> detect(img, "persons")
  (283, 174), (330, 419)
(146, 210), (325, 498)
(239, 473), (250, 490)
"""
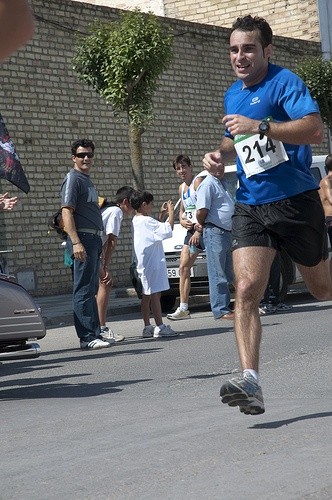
(0, 191), (18, 210)
(61, 139), (115, 349)
(257, 246), (295, 315)
(317, 154), (332, 257)
(129, 189), (179, 338)
(0, 0), (35, 57)
(166, 154), (206, 320)
(201, 14), (332, 415)
(195, 163), (236, 321)
(63, 186), (137, 341)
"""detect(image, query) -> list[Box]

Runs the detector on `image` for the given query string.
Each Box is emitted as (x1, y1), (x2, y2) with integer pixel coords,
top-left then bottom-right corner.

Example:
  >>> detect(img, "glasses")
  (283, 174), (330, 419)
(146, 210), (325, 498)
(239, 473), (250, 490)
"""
(73, 152), (95, 159)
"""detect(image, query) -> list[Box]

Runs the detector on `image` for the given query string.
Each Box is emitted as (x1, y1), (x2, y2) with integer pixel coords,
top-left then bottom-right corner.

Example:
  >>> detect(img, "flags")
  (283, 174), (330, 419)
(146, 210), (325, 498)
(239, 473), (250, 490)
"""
(0, 113), (30, 195)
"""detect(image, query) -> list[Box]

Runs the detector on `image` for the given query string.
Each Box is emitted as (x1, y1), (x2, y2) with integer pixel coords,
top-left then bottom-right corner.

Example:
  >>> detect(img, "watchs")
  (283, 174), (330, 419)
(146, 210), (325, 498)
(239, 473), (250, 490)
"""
(257, 120), (270, 140)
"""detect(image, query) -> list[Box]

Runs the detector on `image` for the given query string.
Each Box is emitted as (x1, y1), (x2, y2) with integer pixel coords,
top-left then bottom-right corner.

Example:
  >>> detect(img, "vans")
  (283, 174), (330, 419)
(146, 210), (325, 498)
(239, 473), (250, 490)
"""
(129, 154), (328, 312)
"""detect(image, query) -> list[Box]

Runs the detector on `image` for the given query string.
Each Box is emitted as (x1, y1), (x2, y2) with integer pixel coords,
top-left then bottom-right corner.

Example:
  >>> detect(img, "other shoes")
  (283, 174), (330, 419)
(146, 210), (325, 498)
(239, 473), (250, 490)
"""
(99, 327), (126, 342)
(273, 302), (293, 311)
(166, 307), (192, 320)
(87, 337), (115, 349)
(257, 304), (277, 316)
(215, 311), (235, 320)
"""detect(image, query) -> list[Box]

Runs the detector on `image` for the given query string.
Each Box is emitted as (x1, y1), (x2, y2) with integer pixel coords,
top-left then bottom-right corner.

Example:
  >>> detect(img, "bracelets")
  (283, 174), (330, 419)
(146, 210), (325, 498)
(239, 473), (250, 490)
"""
(72, 240), (82, 245)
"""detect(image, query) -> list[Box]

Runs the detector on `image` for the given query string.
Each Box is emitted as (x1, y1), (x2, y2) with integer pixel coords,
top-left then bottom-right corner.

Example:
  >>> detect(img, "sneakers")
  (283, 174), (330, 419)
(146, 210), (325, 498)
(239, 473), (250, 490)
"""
(218, 373), (266, 416)
(153, 325), (181, 338)
(142, 326), (154, 338)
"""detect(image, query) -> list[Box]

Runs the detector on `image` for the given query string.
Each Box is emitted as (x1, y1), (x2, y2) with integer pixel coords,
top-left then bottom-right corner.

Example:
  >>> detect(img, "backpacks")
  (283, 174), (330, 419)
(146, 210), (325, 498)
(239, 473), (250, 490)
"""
(47, 196), (120, 240)
